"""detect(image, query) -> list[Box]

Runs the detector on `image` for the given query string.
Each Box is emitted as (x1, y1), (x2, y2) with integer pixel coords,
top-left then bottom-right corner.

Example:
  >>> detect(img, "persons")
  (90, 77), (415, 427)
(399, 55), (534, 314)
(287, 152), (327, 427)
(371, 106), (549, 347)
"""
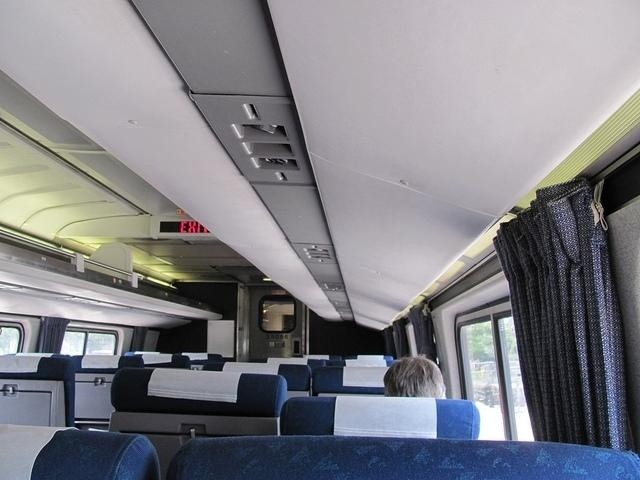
(383, 354), (447, 399)
